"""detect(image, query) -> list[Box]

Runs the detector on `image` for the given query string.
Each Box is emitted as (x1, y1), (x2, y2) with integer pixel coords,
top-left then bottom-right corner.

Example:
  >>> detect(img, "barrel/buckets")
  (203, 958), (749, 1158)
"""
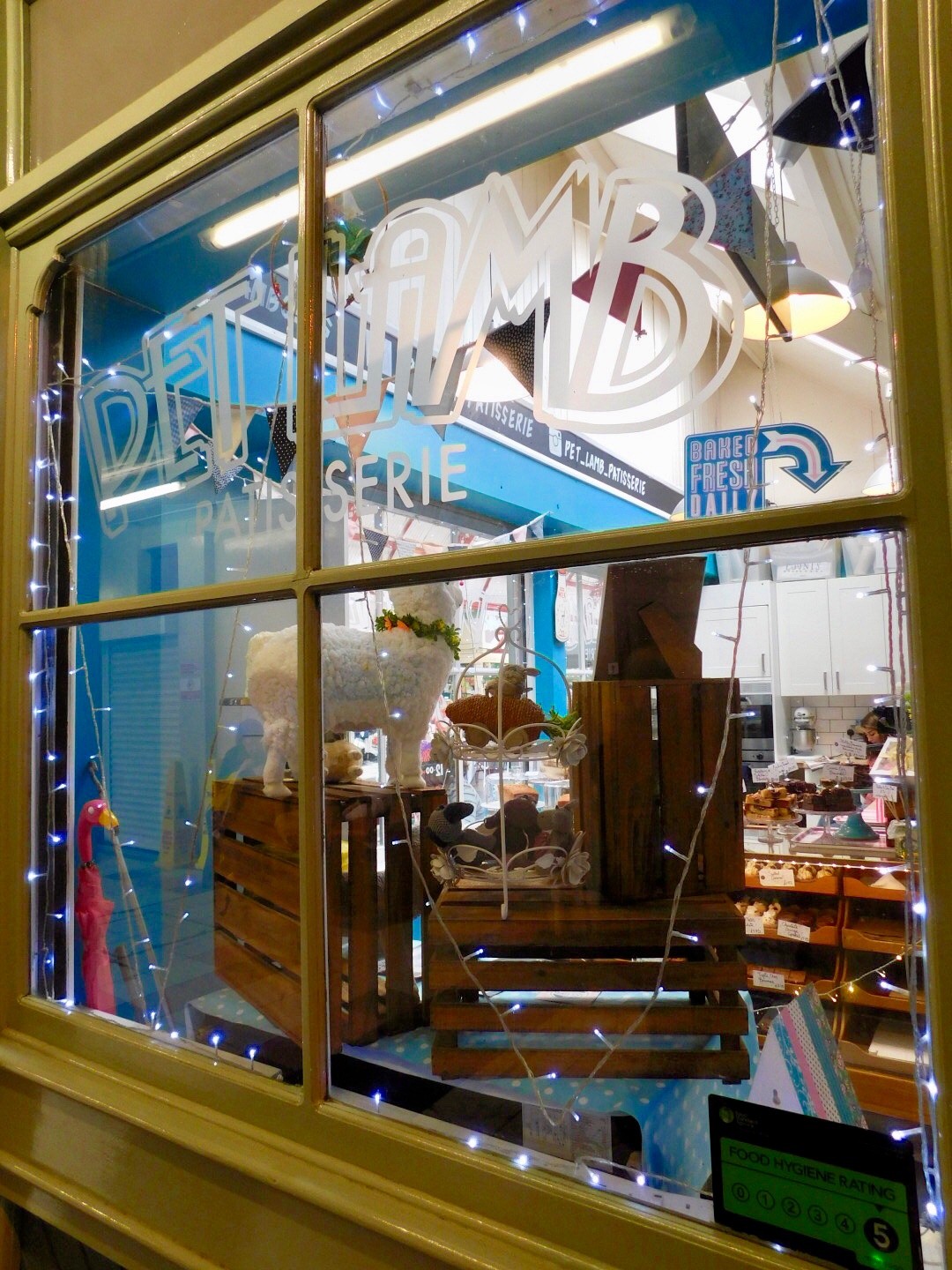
(529, 778), (559, 803)
(485, 776), (528, 807)
(544, 780), (570, 808)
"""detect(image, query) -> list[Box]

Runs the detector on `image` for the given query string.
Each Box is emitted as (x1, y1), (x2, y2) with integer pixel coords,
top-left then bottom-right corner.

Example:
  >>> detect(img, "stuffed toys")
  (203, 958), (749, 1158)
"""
(536, 797), (579, 875)
(482, 794), (538, 869)
(446, 664), (545, 750)
(426, 802), (497, 868)
(245, 581), (466, 800)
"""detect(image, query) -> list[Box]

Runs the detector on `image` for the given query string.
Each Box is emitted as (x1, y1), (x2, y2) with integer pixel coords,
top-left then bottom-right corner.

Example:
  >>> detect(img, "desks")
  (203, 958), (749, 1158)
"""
(182, 940), (756, 1197)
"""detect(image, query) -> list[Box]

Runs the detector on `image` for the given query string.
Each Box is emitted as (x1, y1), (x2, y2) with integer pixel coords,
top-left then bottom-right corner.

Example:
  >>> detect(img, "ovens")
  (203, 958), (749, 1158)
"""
(740, 694), (774, 792)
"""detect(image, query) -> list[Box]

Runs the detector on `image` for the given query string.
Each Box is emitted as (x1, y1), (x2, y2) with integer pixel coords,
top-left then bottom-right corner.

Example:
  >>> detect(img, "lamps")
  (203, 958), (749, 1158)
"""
(458, 346), (531, 404)
(199, 1), (703, 254)
(729, 158), (852, 342)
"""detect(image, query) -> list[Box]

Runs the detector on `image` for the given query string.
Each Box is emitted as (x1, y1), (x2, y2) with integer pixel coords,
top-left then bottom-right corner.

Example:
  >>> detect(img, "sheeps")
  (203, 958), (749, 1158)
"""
(247, 580), (467, 803)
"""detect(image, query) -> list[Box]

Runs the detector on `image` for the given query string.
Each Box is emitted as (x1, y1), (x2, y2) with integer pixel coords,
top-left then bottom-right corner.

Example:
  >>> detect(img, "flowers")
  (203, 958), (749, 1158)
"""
(375, 609), (461, 660)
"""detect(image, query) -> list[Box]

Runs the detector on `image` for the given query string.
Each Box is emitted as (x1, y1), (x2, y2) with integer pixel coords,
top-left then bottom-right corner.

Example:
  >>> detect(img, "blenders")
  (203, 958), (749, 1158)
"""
(791, 707), (817, 751)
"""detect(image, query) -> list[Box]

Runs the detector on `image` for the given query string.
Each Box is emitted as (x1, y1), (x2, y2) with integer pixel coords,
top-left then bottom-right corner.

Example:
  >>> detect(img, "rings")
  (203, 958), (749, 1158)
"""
(869, 799), (871, 802)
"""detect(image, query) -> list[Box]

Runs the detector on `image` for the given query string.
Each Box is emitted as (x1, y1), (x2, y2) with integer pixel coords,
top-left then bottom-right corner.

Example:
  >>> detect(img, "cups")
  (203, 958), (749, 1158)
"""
(377, 846), (385, 871)
(341, 841), (348, 873)
(894, 820), (918, 858)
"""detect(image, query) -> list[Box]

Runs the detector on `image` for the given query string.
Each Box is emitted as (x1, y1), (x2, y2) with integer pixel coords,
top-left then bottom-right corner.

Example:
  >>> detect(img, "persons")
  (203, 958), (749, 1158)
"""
(857, 704), (917, 825)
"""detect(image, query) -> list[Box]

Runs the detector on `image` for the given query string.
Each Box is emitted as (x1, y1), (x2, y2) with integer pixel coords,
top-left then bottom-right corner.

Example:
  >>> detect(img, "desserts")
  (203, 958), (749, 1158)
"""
(744, 764), (874, 820)
(745, 859), (835, 879)
(735, 895), (836, 931)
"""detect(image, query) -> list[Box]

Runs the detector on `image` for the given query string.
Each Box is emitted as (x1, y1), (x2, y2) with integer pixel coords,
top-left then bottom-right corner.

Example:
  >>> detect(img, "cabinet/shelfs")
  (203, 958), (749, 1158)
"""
(741, 853), (932, 1123)
(696, 605), (771, 678)
(430, 605), (591, 919)
(776, 573), (910, 699)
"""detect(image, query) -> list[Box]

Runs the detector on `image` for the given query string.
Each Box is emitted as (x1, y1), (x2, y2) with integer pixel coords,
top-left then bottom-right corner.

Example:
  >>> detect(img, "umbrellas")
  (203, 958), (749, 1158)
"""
(76, 799), (120, 1011)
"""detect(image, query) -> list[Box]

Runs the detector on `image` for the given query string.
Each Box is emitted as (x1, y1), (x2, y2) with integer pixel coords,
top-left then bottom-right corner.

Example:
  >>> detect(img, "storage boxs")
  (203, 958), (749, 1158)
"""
(768, 540), (841, 582)
(715, 546), (771, 582)
(212, 777), (448, 1053)
(574, 679), (745, 903)
(842, 535), (906, 574)
(428, 873), (750, 1086)
